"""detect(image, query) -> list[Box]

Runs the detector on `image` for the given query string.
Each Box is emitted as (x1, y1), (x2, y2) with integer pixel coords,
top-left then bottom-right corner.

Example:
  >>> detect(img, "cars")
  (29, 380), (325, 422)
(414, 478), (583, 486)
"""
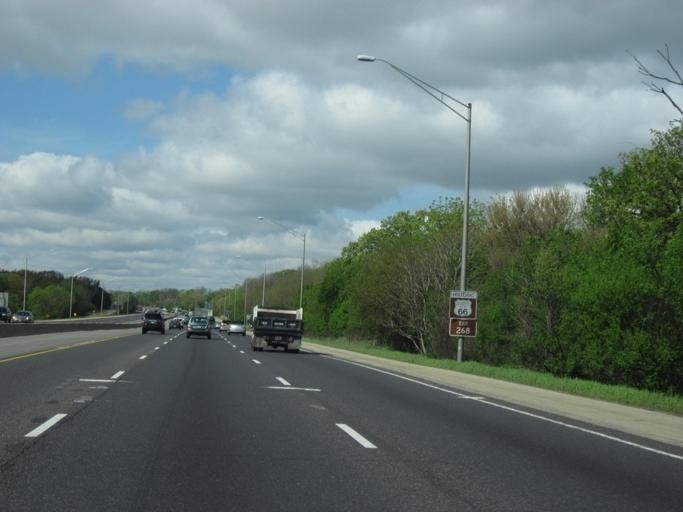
(9, 310), (33, 323)
(219, 319), (230, 332)
(227, 319), (245, 336)
(168, 311), (192, 330)
(214, 320), (221, 329)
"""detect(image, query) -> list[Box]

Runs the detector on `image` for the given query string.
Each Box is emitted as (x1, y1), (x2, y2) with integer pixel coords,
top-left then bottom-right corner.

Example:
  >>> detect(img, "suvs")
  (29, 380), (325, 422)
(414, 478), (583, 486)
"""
(141, 312), (164, 336)
(0, 307), (11, 323)
(186, 316), (211, 340)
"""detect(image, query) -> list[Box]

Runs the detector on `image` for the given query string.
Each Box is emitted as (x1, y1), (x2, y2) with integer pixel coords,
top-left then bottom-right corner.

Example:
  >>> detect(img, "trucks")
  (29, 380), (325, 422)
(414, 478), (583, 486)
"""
(247, 306), (305, 353)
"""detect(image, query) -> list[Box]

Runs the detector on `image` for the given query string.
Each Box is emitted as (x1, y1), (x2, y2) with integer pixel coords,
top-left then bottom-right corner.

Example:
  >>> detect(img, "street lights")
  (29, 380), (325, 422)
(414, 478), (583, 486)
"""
(100, 276), (137, 315)
(220, 252), (267, 318)
(69, 266), (90, 319)
(355, 55), (473, 364)
(256, 216), (306, 309)
